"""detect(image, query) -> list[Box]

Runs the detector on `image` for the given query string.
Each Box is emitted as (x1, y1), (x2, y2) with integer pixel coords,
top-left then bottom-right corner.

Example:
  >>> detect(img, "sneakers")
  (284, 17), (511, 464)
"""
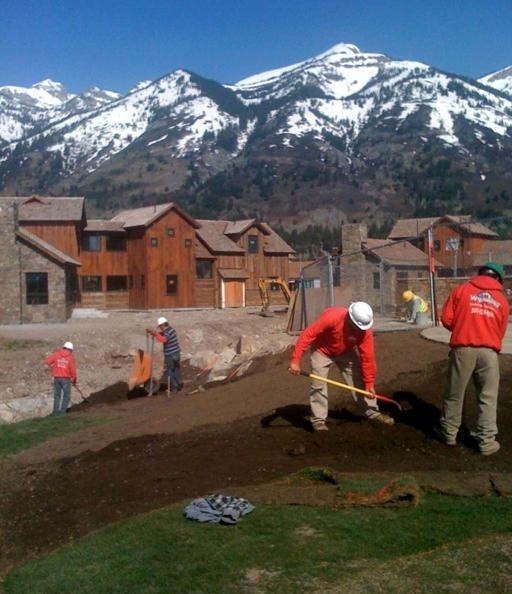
(313, 419), (327, 431)
(482, 442), (499, 456)
(376, 414), (393, 424)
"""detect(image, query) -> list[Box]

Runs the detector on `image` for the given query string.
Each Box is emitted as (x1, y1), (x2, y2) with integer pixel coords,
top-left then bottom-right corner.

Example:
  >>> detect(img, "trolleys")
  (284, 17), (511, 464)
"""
(124, 327), (161, 399)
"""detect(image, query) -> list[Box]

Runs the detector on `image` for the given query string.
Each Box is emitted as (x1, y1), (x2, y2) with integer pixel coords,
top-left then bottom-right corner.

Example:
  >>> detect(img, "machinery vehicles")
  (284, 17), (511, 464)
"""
(254, 276), (295, 319)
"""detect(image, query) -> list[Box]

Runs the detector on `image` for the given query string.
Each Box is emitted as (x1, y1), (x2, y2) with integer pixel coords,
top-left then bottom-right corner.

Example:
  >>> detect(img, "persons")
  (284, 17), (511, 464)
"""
(145, 317), (182, 394)
(290, 301), (396, 432)
(401, 290), (429, 326)
(439, 261), (511, 456)
(44, 342), (77, 416)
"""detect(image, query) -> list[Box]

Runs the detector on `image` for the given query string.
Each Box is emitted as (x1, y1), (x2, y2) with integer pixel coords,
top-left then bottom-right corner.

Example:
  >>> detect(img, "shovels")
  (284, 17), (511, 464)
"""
(287, 367), (426, 412)
(48, 363), (90, 403)
(221, 345), (263, 383)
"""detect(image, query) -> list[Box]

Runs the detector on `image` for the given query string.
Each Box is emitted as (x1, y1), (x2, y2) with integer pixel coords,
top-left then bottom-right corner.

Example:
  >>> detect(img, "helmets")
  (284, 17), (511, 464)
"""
(63, 342), (73, 350)
(479, 261), (504, 283)
(403, 289), (413, 303)
(156, 317), (167, 329)
(349, 302), (373, 331)
